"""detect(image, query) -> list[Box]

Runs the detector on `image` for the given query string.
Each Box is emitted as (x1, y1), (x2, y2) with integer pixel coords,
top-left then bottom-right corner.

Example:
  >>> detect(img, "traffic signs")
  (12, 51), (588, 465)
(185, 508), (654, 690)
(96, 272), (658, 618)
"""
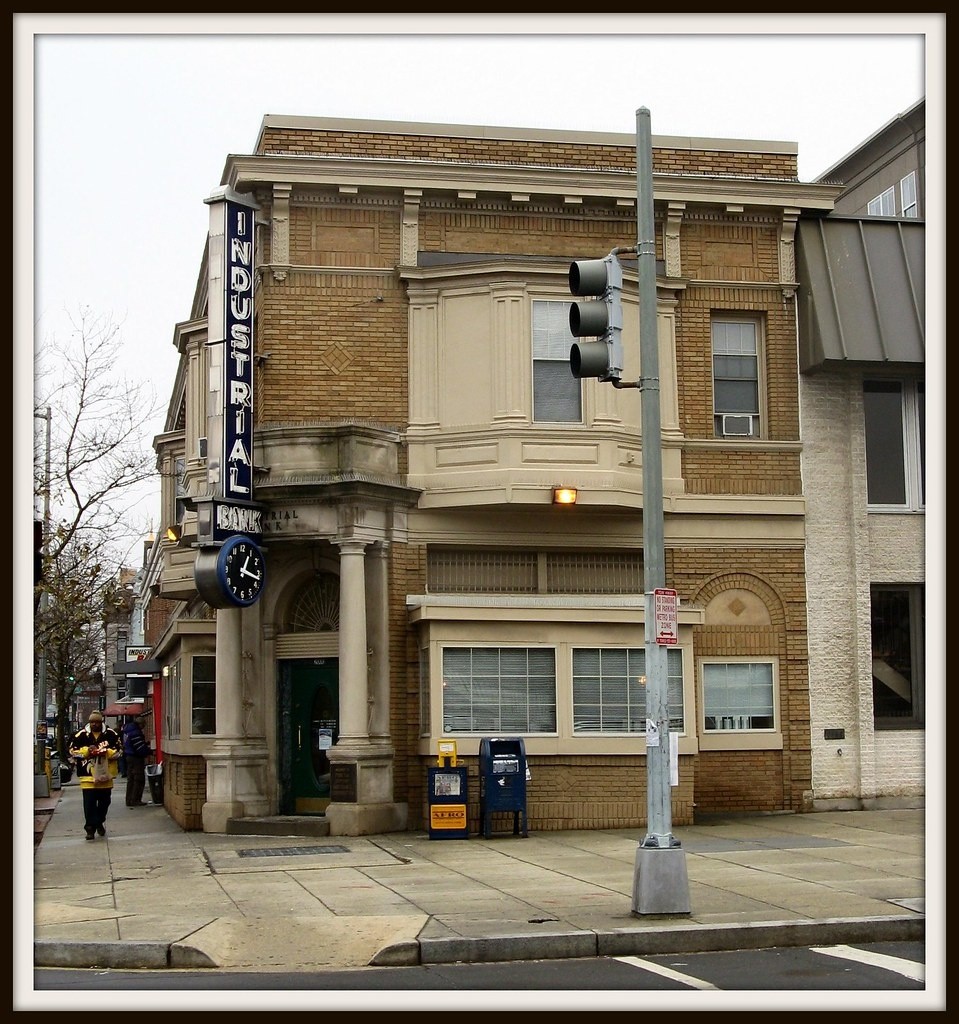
(654, 586), (678, 647)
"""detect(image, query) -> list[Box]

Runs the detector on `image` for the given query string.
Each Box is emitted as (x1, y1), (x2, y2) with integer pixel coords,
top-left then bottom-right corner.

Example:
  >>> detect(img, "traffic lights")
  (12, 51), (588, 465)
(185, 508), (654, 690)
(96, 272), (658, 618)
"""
(565, 254), (638, 391)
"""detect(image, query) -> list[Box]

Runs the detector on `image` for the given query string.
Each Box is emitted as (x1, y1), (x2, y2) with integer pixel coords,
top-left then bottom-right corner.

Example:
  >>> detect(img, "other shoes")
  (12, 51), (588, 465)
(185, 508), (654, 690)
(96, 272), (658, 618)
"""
(85, 833), (95, 840)
(126, 800), (147, 806)
(96, 824), (106, 836)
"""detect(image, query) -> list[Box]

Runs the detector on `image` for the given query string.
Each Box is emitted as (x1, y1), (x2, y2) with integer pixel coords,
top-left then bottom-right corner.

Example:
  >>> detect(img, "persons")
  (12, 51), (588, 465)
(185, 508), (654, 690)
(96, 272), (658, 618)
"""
(106, 717), (154, 806)
(69, 709), (123, 840)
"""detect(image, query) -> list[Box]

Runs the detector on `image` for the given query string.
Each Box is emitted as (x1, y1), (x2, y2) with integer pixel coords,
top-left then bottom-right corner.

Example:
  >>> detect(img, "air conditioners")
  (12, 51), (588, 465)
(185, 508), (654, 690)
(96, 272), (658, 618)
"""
(722, 413), (753, 436)
(197, 437), (209, 459)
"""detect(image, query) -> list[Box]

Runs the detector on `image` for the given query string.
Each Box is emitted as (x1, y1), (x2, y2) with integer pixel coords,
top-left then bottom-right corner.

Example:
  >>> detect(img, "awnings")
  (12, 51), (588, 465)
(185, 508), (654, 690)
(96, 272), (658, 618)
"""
(101, 696), (146, 716)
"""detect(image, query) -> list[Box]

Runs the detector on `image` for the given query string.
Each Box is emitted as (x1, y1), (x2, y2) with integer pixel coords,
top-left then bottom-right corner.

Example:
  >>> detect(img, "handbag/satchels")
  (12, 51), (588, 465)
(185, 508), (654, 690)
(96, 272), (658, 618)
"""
(92, 749), (113, 785)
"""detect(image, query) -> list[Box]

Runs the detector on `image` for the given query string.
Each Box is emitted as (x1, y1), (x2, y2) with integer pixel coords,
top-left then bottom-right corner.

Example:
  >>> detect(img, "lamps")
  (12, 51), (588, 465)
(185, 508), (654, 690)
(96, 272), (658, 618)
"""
(552, 487), (577, 504)
(167, 525), (183, 541)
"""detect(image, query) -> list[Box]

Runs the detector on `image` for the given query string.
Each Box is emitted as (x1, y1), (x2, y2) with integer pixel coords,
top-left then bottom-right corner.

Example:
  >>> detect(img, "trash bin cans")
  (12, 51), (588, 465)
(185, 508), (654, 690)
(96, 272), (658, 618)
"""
(145, 764), (164, 804)
(478, 737), (527, 840)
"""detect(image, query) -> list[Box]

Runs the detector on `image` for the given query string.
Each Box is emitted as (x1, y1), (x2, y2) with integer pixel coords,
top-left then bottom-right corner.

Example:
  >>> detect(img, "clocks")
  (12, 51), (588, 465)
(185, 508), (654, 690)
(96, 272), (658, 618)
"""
(194, 533), (265, 608)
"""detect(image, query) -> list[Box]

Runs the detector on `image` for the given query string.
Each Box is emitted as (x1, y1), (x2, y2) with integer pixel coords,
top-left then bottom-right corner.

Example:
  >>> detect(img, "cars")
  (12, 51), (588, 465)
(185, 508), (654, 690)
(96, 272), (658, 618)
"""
(46, 733), (72, 783)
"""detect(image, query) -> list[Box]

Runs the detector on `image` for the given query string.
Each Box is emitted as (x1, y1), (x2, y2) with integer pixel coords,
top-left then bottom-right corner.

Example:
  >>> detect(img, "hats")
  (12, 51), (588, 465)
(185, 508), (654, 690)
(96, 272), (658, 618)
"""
(89, 710), (102, 722)
(135, 717), (146, 724)
